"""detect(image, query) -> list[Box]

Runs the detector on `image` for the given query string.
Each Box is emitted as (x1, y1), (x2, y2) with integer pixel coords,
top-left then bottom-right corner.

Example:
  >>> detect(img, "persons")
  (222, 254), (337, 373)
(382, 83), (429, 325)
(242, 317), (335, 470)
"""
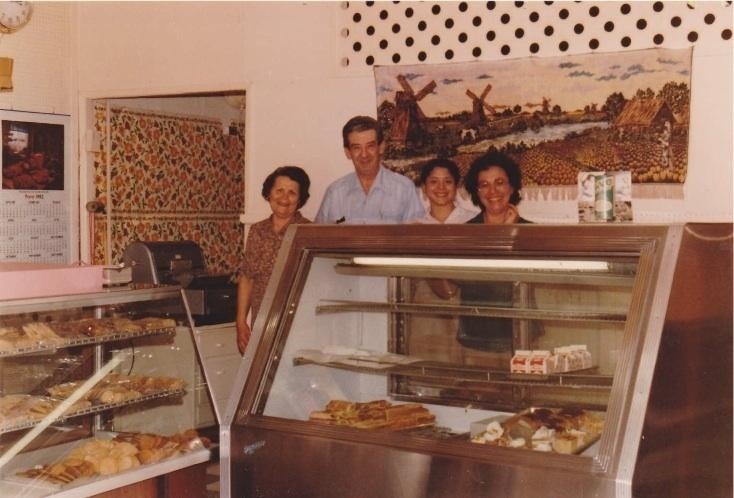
(456, 152), (545, 371)
(314, 114), (425, 224)
(236, 167), (313, 357)
(404, 157), (480, 365)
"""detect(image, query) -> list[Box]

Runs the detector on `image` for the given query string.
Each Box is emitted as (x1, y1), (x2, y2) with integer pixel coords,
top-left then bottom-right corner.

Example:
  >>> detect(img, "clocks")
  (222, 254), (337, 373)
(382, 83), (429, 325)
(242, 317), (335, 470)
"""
(0, 0), (33, 32)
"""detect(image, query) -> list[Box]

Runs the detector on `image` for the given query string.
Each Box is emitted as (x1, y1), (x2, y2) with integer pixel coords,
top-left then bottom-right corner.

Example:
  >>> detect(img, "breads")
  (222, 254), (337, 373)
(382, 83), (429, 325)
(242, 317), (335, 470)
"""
(472, 402), (604, 457)
(0, 313), (212, 485)
(306, 398), (436, 433)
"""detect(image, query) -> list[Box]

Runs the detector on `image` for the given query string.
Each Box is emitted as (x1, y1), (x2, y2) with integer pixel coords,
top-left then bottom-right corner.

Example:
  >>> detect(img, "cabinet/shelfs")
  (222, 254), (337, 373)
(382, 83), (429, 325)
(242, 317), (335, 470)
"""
(0, 286), (221, 498)
(108, 323), (242, 436)
(221, 221), (734, 498)
